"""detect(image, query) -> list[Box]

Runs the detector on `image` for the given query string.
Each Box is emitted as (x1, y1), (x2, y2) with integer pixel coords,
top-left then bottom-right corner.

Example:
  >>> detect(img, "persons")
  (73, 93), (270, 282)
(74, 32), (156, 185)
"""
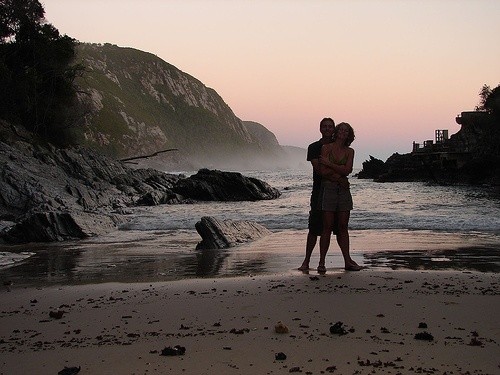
(317, 122), (363, 274)
(297, 117), (361, 270)
